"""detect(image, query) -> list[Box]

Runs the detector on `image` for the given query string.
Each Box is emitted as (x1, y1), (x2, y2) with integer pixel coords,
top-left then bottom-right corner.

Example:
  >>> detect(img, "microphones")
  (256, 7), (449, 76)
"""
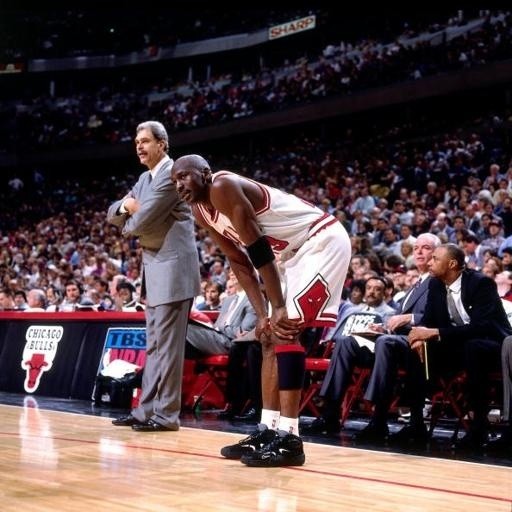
(191, 338), (469, 438)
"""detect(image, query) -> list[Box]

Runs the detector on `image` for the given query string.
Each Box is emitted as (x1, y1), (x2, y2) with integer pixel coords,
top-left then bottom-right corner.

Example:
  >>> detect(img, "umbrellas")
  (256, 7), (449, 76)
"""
(385, 424), (427, 445)
(452, 438), (478, 450)
(131, 420), (171, 431)
(112, 415), (145, 425)
(304, 417), (330, 435)
(111, 372), (141, 387)
(352, 423), (390, 441)
(218, 403), (241, 419)
(482, 436), (512, 454)
(233, 406), (260, 421)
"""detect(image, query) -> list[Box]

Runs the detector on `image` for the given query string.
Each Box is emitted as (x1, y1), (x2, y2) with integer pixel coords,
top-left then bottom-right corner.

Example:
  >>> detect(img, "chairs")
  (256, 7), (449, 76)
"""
(223, 424), (280, 463)
(242, 429), (305, 466)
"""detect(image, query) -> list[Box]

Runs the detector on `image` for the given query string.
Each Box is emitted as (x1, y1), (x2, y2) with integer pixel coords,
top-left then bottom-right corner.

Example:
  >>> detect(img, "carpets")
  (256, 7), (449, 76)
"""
(415, 279), (423, 289)
(447, 288), (463, 326)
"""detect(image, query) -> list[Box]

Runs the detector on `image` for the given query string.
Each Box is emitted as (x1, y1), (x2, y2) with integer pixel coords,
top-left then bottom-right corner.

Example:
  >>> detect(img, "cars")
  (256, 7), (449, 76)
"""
(193, 396), (205, 413)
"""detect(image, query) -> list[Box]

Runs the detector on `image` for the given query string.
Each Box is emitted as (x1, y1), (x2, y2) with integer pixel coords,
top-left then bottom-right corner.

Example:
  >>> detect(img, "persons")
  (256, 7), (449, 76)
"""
(299, 233), (440, 446)
(109, 269), (267, 389)
(13, 3), (511, 143)
(325, 275), (398, 339)
(2, 176), (253, 312)
(384, 242), (512, 452)
(107, 119), (202, 432)
(172, 154), (352, 466)
(236, 144), (512, 311)
